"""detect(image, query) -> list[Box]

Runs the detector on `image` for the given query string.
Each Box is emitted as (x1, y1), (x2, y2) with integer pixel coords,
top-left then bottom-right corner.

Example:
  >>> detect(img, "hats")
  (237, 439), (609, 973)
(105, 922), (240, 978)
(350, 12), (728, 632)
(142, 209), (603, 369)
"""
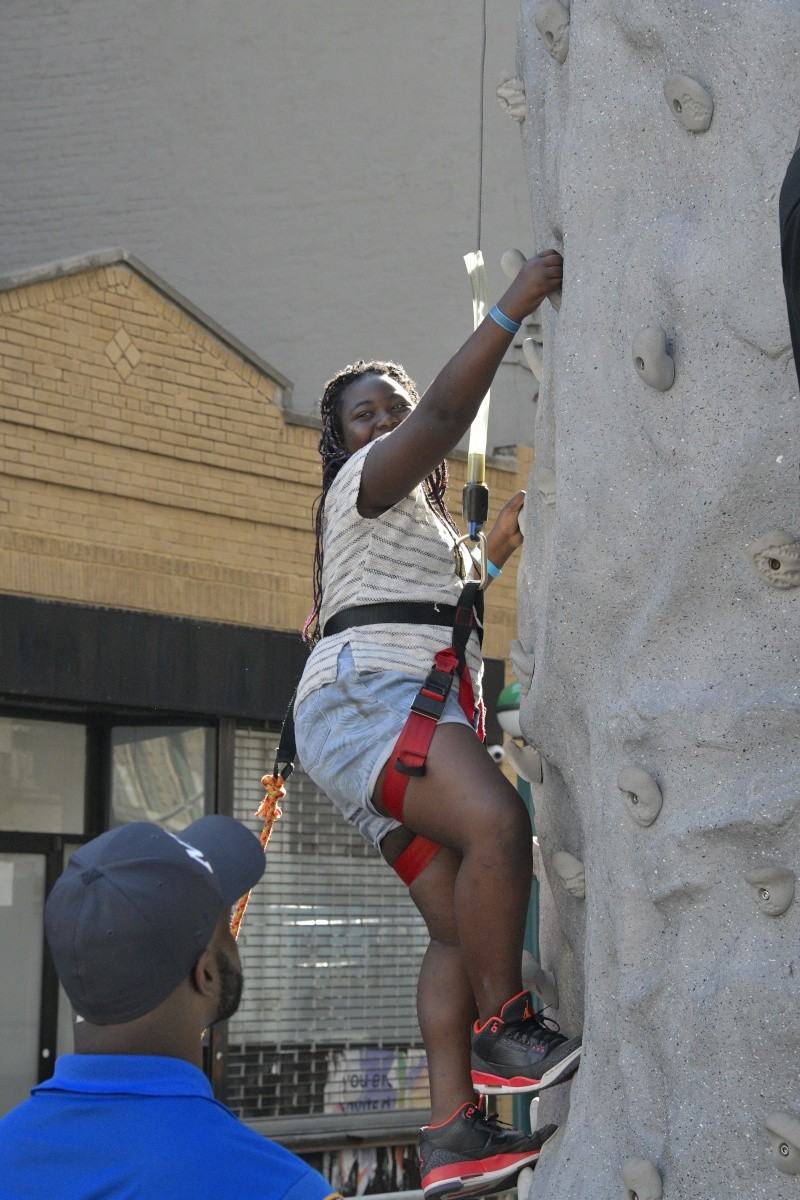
(45, 813), (267, 1023)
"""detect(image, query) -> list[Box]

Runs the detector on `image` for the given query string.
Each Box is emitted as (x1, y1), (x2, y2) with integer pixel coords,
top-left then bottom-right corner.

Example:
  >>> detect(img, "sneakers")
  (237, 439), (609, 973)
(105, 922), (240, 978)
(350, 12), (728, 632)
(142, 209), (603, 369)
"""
(470, 989), (583, 1094)
(418, 1094), (558, 1200)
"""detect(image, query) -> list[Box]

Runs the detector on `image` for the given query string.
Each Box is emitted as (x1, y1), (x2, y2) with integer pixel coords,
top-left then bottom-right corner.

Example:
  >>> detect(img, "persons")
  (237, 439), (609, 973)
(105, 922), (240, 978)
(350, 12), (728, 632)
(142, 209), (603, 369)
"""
(0, 814), (344, 1199)
(290, 248), (585, 1200)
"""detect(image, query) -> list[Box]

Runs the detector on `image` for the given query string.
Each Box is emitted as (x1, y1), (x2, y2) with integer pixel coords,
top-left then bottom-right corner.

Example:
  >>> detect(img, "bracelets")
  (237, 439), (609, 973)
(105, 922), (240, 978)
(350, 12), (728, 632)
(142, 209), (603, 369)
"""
(489, 303), (521, 334)
(471, 543), (502, 580)
